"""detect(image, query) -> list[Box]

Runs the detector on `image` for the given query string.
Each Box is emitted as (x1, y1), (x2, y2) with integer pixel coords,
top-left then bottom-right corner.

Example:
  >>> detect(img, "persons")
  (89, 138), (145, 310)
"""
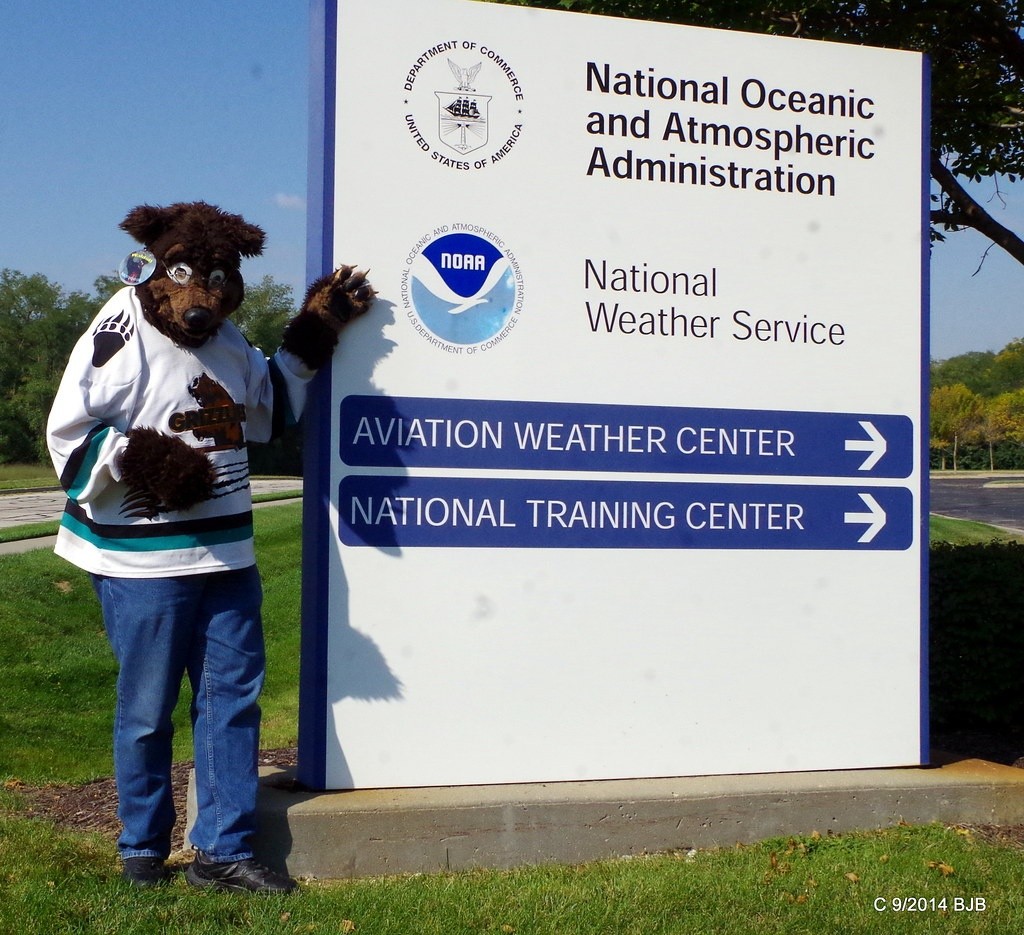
(43, 203), (377, 897)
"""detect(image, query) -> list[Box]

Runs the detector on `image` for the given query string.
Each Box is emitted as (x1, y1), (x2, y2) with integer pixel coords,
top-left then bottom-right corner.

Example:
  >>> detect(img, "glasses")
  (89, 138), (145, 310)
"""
(161, 256), (225, 293)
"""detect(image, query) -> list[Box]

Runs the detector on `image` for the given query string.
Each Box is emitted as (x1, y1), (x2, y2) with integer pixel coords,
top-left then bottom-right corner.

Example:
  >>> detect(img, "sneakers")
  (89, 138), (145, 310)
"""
(188, 845), (298, 898)
(120, 856), (163, 893)
